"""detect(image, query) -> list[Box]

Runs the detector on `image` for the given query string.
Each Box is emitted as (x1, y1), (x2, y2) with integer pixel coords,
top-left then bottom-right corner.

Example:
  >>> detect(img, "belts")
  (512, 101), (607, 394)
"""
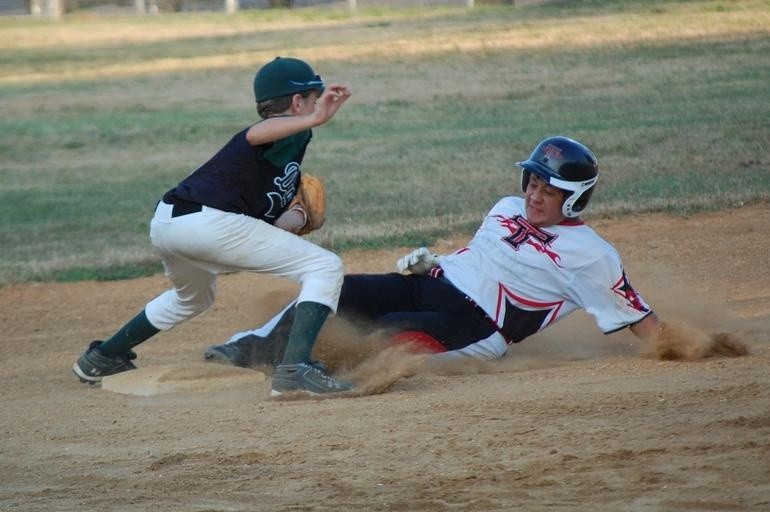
(154, 200), (202, 217)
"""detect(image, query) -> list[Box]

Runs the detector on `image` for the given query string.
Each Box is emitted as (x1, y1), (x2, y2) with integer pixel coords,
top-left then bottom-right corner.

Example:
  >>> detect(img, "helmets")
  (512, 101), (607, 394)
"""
(521, 136), (599, 218)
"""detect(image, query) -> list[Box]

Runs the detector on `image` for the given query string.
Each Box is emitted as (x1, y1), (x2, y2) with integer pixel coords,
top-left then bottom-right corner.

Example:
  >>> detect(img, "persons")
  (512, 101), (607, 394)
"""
(73, 57), (356, 395)
(203, 135), (749, 384)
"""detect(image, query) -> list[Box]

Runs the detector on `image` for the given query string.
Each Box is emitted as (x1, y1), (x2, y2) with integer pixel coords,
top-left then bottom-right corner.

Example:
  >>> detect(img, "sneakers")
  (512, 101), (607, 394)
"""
(270, 363), (354, 397)
(205, 343), (250, 368)
(72, 341), (137, 385)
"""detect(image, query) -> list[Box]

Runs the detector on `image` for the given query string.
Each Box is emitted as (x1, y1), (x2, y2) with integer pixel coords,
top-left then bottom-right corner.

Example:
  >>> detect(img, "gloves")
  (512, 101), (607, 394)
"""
(397, 247), (439, 275)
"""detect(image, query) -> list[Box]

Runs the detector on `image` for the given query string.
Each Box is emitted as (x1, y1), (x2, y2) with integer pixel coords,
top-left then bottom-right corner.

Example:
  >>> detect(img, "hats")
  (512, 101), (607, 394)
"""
(254, 59), (326, 103)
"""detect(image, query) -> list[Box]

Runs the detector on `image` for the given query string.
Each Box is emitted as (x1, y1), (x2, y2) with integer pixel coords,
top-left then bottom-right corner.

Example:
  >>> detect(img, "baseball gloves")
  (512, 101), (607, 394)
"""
(288, 172), (326, 236)
